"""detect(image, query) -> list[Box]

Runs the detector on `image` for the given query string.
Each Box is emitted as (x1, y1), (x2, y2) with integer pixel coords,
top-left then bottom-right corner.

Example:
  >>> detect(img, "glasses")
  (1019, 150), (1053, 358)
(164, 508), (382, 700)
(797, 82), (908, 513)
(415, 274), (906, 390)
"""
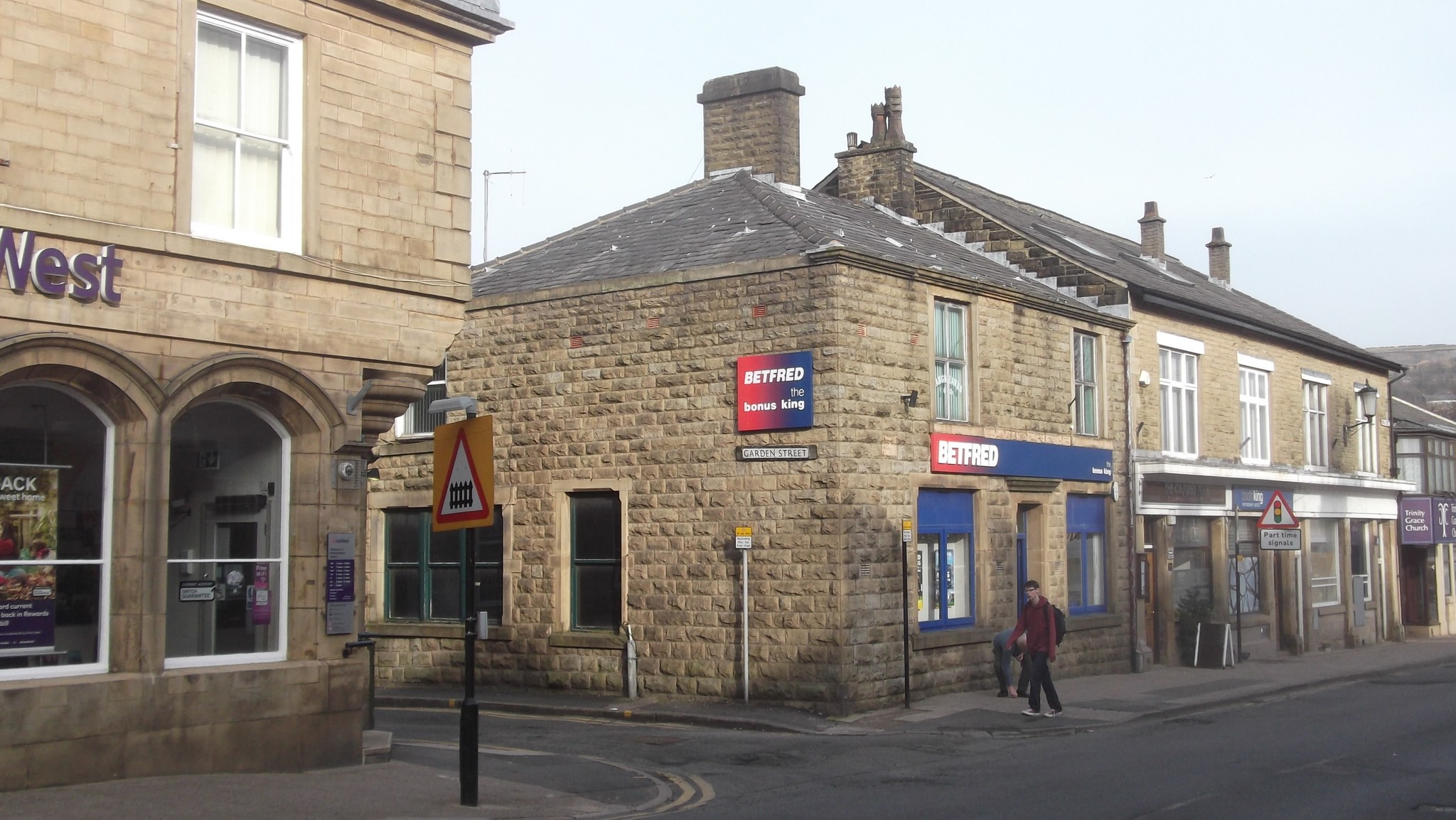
(1024, 589), (1037, 593)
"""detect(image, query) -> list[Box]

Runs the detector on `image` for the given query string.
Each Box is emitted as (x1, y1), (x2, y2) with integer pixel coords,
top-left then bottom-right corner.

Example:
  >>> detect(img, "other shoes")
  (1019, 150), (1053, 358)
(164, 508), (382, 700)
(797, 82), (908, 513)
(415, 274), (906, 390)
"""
(998, 690), (1008, 697)
(1016, 689), (1029, 697)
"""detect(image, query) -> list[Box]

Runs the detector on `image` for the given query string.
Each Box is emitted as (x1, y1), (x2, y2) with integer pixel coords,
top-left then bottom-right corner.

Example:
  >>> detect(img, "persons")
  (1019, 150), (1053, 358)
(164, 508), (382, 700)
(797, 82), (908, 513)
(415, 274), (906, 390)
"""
(1004, 579), (1063, 718)
(992, 626), (1029, 698)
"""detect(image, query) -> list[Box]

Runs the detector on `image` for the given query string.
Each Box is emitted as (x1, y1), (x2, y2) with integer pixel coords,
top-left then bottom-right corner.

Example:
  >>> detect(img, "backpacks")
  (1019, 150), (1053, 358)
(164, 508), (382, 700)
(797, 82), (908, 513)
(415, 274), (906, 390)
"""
(1043, 603), (1066, 646)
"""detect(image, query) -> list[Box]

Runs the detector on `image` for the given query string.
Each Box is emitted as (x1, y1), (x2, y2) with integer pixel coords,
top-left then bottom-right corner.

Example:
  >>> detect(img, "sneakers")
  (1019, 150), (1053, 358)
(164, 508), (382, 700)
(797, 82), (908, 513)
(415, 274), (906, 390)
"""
(1044, 708), (1062, 718)
(1022, 708), (1040, 716)
